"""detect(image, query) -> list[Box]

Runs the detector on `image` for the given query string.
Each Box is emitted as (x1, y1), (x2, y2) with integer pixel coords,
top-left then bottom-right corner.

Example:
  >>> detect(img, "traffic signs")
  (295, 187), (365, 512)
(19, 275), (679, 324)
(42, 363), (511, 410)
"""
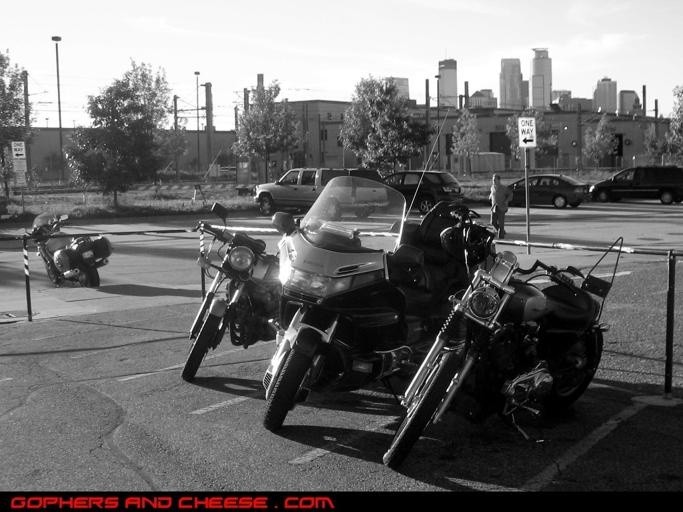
(12, 141), (25, 158)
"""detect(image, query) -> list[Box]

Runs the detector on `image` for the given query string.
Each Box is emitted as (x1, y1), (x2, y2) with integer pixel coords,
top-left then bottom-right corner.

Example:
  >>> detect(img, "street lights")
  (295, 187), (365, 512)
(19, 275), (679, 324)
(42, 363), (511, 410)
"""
(576, 103), (602, 170)
(195, 72), (200, 174)
(52, 36), (65, 185)
(519, 118), (537, 150)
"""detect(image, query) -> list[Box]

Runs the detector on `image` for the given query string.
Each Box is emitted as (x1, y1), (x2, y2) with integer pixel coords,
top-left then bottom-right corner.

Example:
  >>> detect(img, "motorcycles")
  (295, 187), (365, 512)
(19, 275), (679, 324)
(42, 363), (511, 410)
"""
(261, 173), (506, 433)
(383, 216), (623, 471)
(181, 203), (280, 383)
(28, 214), (112, 287)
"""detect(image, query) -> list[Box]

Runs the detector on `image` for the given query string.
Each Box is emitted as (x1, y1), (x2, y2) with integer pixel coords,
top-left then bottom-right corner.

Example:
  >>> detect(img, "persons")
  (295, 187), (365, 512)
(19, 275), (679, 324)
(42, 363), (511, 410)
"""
(488, 173), (514, 240)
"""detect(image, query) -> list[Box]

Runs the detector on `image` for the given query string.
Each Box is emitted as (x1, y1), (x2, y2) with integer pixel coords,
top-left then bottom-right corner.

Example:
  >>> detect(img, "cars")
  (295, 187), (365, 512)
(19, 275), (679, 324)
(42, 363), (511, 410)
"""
(488, 172), (592, 208)
(588, 164), (683, 205)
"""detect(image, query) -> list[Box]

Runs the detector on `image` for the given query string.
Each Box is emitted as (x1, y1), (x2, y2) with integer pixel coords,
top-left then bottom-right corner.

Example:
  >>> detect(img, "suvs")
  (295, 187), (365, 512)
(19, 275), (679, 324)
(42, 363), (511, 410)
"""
(381, 169), (464, 215)
(255, 168), (382, 215)
